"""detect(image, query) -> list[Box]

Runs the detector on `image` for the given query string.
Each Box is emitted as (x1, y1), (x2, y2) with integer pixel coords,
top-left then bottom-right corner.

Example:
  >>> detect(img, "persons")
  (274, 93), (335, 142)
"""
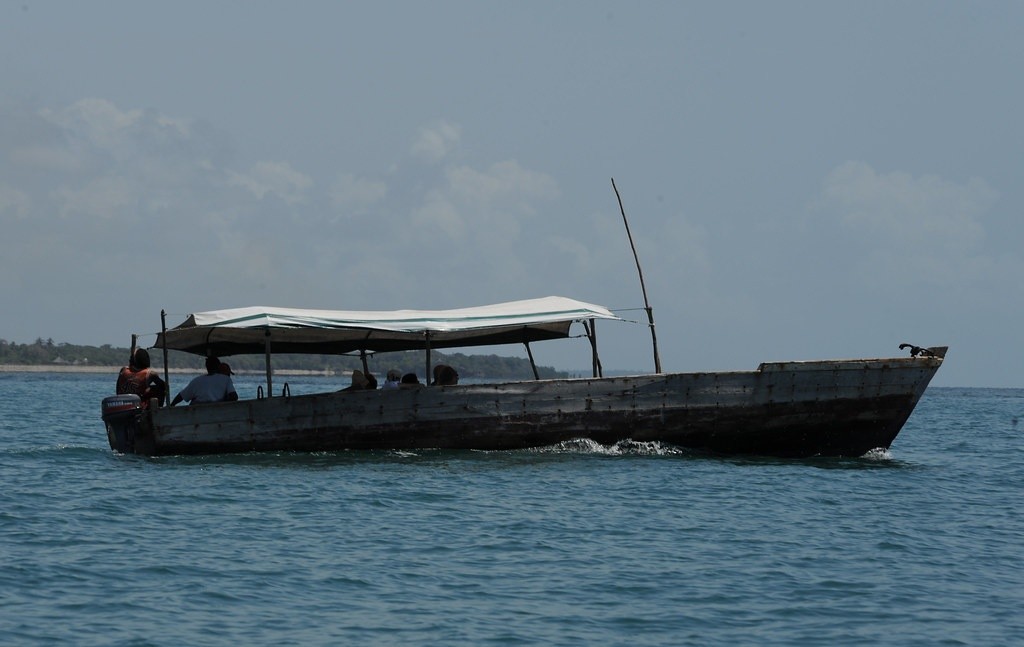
(367, 366), (458, 389)
(172, 357), (239, 406)
(116, 349), (166, 407)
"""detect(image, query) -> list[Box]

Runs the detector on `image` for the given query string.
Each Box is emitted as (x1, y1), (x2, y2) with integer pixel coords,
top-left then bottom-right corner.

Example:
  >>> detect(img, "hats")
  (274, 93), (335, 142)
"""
(219, 363), (235, 375)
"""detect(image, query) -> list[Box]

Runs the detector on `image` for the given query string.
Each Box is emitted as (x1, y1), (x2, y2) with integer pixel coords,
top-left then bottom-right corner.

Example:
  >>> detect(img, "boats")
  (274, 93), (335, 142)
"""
(100, 296), (949, 456)
